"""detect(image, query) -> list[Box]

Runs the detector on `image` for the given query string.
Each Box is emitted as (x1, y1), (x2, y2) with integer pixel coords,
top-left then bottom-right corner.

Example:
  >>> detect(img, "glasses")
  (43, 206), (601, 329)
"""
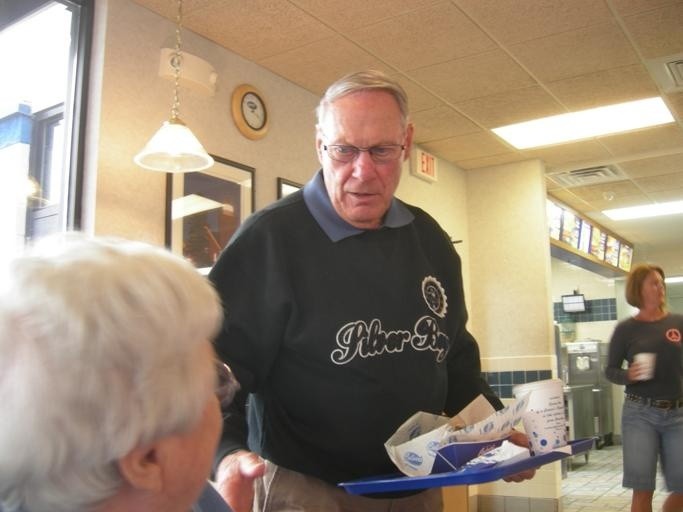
(217, 361), (241, 408)
(324, 144), (405, 163)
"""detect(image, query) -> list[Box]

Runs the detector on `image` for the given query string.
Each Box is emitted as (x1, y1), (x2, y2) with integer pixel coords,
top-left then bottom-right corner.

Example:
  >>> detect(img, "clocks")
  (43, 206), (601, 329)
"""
(232, 84), (271, 140)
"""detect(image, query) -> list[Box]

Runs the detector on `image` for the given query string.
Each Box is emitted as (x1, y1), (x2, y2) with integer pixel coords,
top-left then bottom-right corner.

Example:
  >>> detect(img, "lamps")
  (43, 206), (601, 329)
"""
(135, 0), (214, 173)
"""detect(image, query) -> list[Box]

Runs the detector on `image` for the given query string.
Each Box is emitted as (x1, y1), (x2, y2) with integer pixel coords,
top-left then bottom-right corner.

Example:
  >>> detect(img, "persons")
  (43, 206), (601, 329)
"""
(0, 232), (239, 512)
(604, 261), (683, 511)
(204, 69), (542, 510)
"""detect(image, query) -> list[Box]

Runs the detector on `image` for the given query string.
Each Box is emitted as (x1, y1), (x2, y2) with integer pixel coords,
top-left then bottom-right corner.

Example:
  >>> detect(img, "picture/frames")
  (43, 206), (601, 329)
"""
(276, 178), (305, 198)
(165, 153), (256, 281)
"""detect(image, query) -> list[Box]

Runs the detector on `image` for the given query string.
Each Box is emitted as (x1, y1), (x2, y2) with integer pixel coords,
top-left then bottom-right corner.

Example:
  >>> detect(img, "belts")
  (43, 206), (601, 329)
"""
(625, 393), (683, 410)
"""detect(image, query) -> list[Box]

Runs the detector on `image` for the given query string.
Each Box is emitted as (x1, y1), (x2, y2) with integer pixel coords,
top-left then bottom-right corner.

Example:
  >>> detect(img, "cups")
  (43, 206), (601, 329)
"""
(512, 377), (569, 457)
(633, 352), (657, 382)
